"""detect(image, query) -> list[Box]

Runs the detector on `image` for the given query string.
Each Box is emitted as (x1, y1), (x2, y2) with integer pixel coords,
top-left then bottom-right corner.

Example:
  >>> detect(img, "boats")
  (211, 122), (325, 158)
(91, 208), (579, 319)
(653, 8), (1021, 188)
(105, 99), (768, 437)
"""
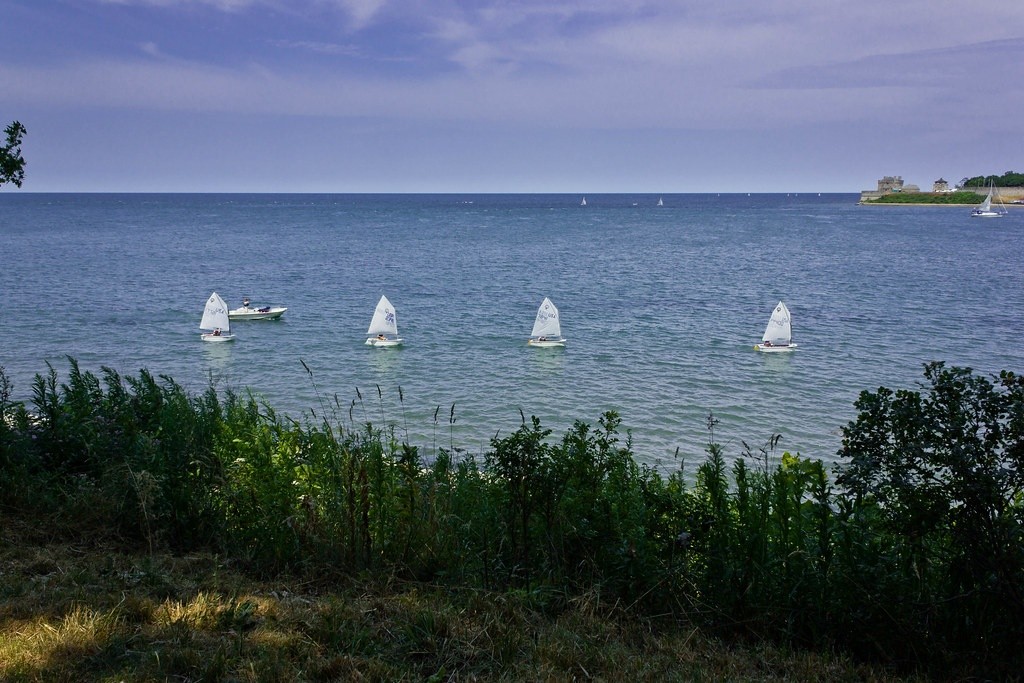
(228, 298), (287, 321)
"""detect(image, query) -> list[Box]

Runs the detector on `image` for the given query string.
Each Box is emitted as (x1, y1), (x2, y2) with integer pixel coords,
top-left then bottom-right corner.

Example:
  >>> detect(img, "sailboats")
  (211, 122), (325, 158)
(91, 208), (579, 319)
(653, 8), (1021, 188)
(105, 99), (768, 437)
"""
(199, 292), (236, 342)
(526, 297), (566, 347)
(754, 301), (797, 354)
(365, 295), (403, 347)
(972, 175), (1008, 218)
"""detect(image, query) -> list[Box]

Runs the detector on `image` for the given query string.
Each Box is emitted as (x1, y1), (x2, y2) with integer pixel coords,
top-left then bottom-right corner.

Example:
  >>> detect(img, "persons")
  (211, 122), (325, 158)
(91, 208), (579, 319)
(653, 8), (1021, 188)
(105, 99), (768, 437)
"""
(244, 298), (249, 306)
(212, 328), (221, 336)
(977, 211), (981, 214)
(764, 341), (771, 347)
(378, 335), (384, 340)
(538, 336), (545, 341)
